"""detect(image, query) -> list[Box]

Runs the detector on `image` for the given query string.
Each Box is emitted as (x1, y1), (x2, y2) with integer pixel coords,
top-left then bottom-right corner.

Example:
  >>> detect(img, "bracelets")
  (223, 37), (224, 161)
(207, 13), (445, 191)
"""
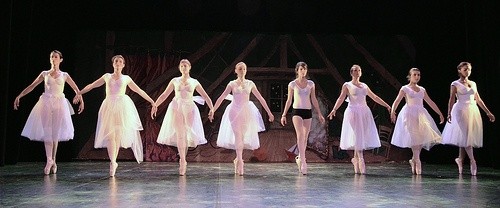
(448, 114), (451, 115)
(282, 115), (286, 117)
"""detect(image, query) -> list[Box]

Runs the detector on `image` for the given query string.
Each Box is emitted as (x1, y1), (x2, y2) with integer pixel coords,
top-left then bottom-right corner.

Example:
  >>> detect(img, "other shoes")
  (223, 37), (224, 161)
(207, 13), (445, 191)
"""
(359, 158), (365, 174)
(409, 159), (416, 174)
(455, 158), (464, 174)
(44, 158), (53, 175)
(301, 159), (307, 175)
(234, 158), (238, 174)
(110, 162), (117, 177)
(237, 159), (245, 176)
(178, 159), (186, 176)
(295, 156), (301, 172)
(52, 162), (57, 173)
(470, 160), (477, 176)
(416, 160), (421, 174)
(351, 158), (359, 173)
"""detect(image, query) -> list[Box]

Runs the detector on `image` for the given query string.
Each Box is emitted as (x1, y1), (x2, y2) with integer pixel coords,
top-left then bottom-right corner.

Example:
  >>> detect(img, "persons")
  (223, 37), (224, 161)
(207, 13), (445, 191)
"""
(73, 54), (157, 177)
(390, 68), (444, 174)
(150, 59), (214, 176)
(14, 50), (84, 174)
(207, 62), (274, 175)
(440, 61), (495, 175)
(327, 64), (396, 174)
(280, 62), (325, 174)
